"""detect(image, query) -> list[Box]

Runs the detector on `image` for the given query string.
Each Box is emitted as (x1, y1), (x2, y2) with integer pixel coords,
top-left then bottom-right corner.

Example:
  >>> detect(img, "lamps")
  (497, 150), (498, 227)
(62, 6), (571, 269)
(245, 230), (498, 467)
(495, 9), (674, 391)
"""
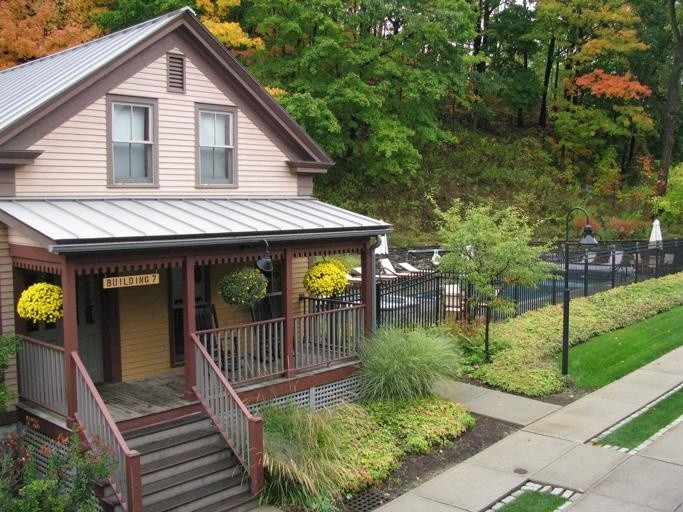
(256, 237), (274, 273)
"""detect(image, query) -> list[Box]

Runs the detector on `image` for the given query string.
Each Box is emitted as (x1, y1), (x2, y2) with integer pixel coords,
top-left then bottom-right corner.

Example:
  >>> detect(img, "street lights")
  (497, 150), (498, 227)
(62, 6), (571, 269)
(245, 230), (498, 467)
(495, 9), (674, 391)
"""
(561, 204), (599, 380)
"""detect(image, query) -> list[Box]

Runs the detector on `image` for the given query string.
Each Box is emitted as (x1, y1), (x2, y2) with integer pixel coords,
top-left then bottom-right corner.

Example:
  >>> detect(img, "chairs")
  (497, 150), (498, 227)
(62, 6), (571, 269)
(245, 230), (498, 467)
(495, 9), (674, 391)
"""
(195, 304), (241, 371)
(250, 296), (281, 363)
(347, 258), (434, 283)
